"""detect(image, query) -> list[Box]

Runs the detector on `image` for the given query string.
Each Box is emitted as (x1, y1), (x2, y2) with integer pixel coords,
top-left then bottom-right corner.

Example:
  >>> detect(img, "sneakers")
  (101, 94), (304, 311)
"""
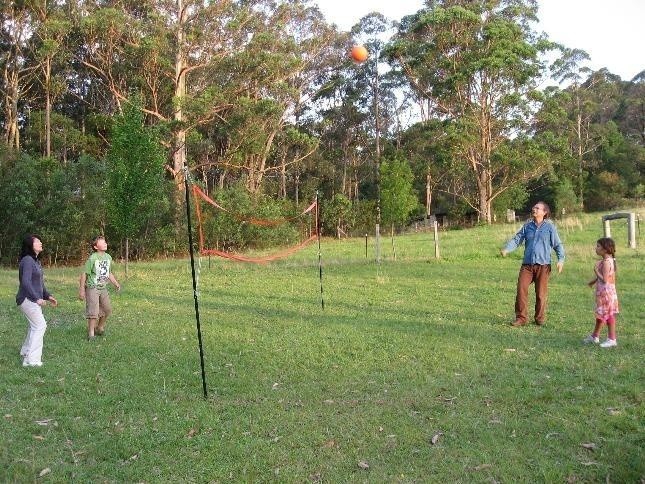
(584, 335), (617, 347)
(88, 329), (104, 341)
(511, 318), (544, 326)
(21, 352), (43, 367)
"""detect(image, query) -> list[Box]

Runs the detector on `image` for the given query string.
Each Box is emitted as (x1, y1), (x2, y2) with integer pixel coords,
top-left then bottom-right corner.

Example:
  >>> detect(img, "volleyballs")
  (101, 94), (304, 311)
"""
(350, 46), (369, 63)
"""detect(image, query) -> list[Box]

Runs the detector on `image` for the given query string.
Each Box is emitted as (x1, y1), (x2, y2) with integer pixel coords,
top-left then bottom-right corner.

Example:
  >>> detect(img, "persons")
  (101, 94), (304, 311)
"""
(583, 237), (620, 348)
(499, 200), (565, 325)
(14, 234), (57, 367)
(79, 235), (122, 340)
